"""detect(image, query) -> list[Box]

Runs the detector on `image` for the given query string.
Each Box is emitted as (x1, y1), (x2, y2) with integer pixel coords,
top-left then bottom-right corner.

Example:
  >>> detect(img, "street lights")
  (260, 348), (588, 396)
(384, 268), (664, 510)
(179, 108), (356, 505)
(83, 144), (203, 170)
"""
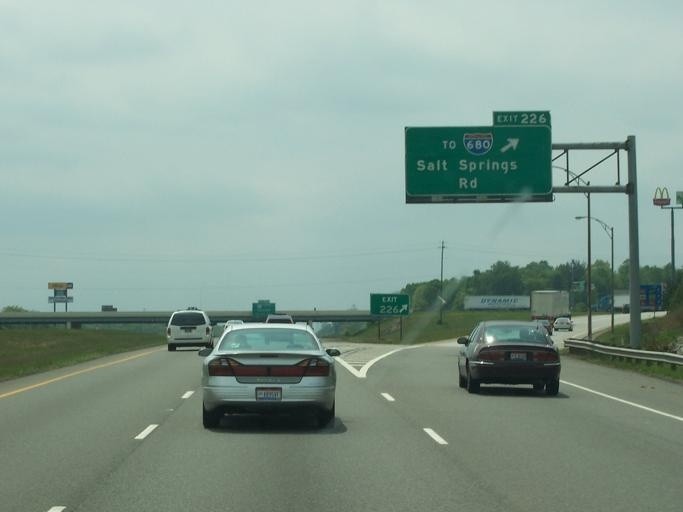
(573, 215), (614, 337)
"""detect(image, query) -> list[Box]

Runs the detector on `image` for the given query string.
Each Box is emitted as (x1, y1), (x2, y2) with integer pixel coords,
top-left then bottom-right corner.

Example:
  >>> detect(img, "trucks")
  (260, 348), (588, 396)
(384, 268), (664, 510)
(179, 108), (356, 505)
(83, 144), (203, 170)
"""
(102, 305), (117, 311)
(590, 288), (630, 314)
(530, 290), (571, 319)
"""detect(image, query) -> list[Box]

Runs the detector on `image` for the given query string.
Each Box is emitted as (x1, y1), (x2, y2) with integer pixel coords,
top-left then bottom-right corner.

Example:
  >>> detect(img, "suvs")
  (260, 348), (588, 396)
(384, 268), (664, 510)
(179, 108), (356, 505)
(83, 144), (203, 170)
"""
(166, 309), (213, 351)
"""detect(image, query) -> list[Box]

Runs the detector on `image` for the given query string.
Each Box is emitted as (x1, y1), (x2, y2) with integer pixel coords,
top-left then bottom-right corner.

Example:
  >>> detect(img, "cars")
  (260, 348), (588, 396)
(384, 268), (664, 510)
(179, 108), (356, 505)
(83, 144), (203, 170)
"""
(196, 322), (340, 425)
(456, 318), (561, 395)
(533, 317), (574, 336)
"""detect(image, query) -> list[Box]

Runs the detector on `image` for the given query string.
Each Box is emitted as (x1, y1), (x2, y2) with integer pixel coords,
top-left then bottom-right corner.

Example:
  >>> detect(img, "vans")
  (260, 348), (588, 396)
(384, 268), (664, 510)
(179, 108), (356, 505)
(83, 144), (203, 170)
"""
(223, 320), (244, 333)
(263, 313), (295, 346)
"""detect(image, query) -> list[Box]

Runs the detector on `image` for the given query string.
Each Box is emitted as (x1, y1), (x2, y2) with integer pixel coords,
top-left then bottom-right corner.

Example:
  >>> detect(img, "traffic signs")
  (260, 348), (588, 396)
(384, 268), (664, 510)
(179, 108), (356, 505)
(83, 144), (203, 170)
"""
(370, 294), (409, 317)
(493, 110), (550, 126)
(404, 126), (552, 196)
(252, 303), (274, 318)
